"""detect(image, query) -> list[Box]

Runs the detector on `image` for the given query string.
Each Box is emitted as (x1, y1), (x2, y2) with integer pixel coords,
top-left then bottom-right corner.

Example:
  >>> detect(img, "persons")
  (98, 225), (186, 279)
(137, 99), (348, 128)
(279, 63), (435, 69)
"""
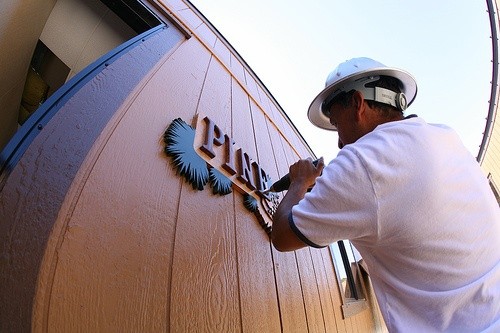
(271, 57), (500, 333)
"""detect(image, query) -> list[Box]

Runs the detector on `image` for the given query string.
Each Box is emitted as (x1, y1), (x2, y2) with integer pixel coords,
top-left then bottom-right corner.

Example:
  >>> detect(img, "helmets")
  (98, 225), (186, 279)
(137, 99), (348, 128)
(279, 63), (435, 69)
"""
(307, 57), (417, 131)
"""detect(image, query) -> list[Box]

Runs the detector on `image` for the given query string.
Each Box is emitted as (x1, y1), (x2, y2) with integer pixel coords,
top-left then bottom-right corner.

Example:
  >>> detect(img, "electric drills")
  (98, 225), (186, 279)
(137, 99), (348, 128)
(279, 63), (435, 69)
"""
(262, 159), (324, 195)
